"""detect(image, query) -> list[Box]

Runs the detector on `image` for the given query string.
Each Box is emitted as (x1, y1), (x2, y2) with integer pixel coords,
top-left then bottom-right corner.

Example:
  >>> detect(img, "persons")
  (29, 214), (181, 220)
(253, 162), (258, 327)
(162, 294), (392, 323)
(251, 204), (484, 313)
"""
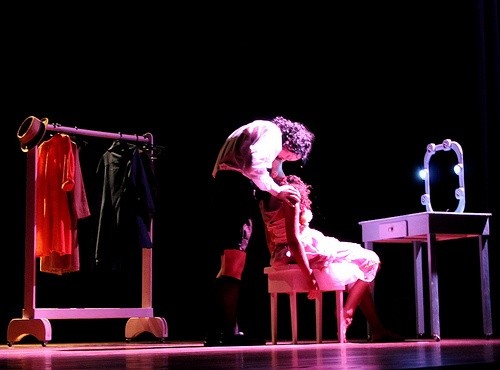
(204, 116), (315, 346)
(269, 157), (407, 343)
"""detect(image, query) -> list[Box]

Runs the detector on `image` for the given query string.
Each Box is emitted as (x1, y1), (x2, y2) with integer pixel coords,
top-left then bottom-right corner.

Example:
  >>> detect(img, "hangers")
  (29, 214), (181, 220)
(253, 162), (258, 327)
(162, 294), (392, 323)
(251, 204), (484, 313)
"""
(40, 123), (164, 159)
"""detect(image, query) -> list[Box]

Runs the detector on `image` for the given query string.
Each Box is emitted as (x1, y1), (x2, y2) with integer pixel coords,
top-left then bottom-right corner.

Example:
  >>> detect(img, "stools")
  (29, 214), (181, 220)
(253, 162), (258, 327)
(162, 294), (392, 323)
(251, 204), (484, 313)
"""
(263, 264), (344, 344)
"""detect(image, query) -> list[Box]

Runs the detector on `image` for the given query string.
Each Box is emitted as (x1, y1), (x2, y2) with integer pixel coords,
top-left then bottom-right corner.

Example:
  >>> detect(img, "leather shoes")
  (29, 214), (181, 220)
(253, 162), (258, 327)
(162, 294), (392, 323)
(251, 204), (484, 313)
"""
(217, 331), (266, 348)
(202, 327), (219, 346)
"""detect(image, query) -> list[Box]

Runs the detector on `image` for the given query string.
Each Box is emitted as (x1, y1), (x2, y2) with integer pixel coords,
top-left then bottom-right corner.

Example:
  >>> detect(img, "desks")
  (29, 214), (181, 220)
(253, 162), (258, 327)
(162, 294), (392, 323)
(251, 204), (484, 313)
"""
(358, 211), (492, 341)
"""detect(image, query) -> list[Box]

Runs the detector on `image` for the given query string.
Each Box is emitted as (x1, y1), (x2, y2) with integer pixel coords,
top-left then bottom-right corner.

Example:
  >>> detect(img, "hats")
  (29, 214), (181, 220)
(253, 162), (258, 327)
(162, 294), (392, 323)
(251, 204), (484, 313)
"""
(17, 116), (49, 153)
